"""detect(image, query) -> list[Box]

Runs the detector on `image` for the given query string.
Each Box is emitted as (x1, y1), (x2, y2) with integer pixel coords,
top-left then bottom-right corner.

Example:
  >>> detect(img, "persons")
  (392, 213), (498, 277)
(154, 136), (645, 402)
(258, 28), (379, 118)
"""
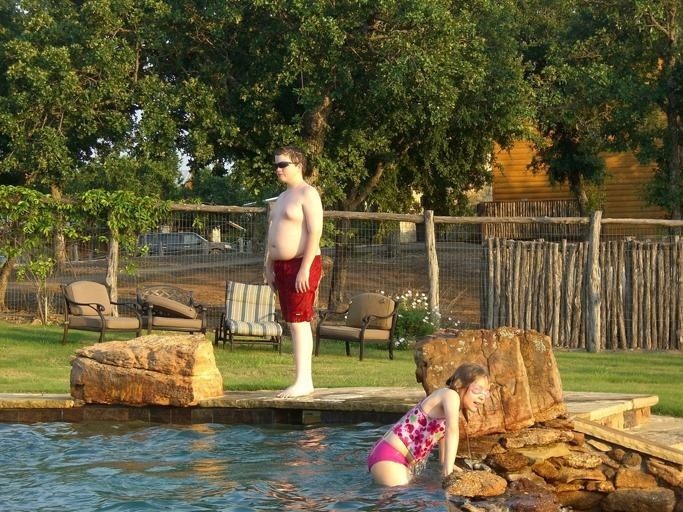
(262, 147), (323, 400)
(366, 360), (491, 489)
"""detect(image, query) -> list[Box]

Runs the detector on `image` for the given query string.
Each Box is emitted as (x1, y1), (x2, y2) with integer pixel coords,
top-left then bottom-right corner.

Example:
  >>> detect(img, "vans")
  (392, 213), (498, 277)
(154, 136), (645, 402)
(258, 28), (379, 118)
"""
(134, 231), (231, 256)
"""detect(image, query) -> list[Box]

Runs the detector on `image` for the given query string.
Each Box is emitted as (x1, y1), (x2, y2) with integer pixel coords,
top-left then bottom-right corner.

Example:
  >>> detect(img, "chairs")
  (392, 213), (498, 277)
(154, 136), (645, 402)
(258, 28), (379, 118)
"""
(215, 278), (283, 354)
(315, 292), (400, 361)
(59, 280), (143, 345)
(134, 284), (206, 335)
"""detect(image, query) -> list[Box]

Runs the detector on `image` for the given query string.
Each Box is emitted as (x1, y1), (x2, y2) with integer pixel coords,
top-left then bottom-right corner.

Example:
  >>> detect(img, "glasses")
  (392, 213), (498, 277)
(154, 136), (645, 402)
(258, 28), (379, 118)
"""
(273, 161), (297, 168)
(464, 380), (491, 400)
